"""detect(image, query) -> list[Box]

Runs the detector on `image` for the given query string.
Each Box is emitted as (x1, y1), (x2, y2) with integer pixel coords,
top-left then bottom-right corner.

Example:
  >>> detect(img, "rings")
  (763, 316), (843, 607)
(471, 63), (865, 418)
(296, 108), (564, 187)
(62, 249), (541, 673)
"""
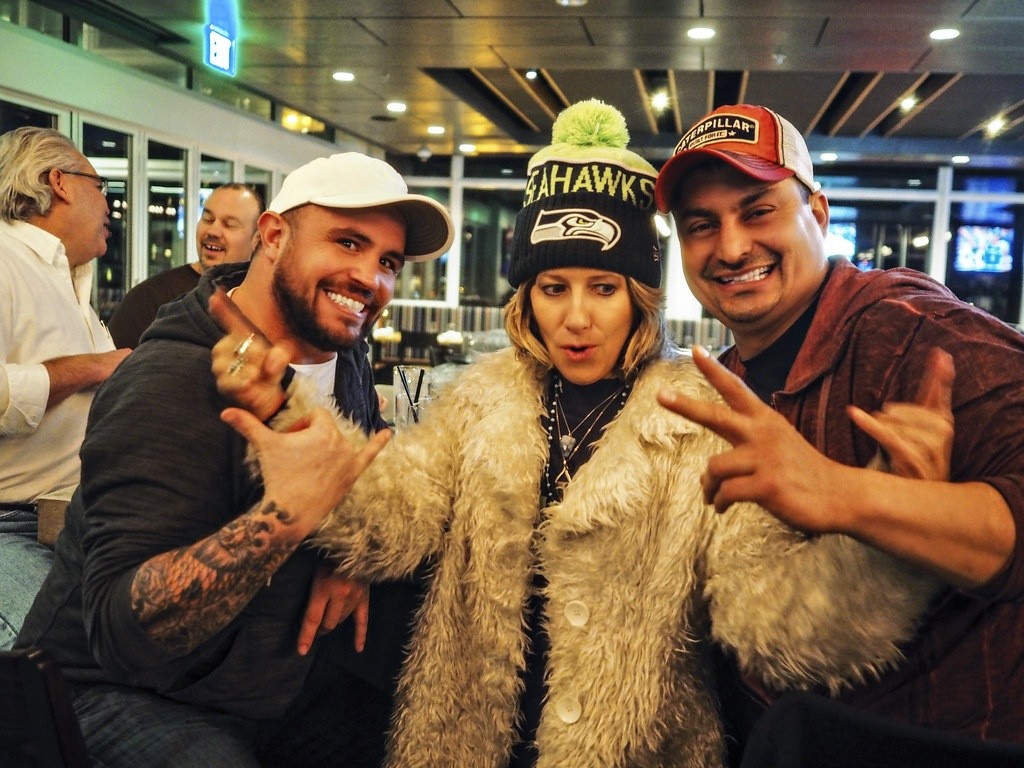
(227, 332), (256, 355)
(226, 358), (249, 378)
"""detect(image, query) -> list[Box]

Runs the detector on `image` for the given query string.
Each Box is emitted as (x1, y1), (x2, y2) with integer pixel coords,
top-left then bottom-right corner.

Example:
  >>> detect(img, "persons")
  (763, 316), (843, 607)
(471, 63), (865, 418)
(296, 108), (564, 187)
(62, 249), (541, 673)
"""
(654, 101), (1024, 768)
(0, 123), (458, 767)
(207, 96), (957, 768)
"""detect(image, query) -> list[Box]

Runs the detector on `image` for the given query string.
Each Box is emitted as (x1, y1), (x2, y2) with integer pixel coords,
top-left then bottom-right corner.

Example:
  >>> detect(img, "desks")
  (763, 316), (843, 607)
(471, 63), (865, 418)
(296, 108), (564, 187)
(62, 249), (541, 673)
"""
(389, 356), (433, 427)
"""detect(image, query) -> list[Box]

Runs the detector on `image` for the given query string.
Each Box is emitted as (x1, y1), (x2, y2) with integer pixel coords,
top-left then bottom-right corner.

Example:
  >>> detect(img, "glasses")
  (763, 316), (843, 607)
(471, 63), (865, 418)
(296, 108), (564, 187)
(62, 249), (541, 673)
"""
(41, 171), (109, 196)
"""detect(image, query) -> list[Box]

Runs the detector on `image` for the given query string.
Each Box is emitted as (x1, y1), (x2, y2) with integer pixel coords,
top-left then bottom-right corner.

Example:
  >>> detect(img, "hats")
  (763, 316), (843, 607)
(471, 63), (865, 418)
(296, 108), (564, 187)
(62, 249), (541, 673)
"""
(507, 98), (662, 289)
(269, 152), (455, 262)
(654, 103), (816, 213)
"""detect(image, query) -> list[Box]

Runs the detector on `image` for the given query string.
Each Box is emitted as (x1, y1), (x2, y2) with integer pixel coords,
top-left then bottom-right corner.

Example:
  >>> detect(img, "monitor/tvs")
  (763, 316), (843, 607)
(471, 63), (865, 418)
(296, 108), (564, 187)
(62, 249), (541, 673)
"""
(954, 226), (1015, 272)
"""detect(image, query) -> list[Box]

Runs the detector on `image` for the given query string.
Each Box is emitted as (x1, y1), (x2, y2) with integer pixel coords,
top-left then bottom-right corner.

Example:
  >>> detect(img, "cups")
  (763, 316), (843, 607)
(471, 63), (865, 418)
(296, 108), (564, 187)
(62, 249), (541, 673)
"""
(393, 365), (432, 425)
(396, 393), (432, 436)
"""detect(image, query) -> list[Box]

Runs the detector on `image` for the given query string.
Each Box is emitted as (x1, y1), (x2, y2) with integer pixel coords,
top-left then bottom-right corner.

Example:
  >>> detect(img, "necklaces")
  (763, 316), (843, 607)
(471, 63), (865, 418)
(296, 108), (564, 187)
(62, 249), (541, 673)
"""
(547, 377), (625, 484)
